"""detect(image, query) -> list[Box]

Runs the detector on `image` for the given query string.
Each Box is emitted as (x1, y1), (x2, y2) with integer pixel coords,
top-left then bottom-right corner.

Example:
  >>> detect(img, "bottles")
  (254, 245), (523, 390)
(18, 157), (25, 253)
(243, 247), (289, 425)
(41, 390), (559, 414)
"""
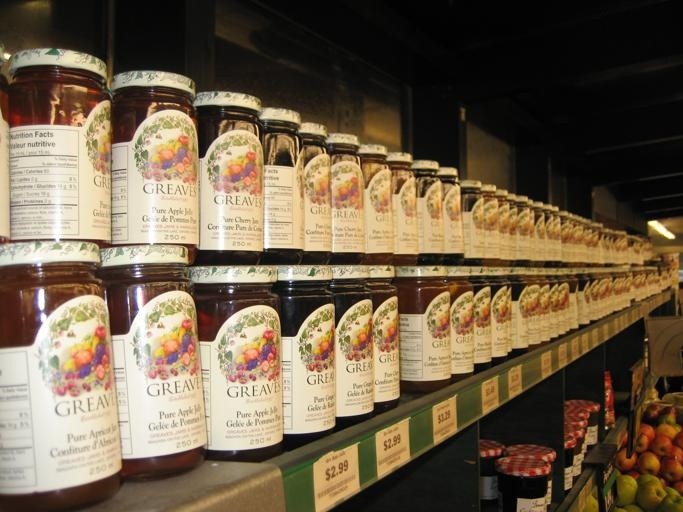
(604, 372), (616, 430)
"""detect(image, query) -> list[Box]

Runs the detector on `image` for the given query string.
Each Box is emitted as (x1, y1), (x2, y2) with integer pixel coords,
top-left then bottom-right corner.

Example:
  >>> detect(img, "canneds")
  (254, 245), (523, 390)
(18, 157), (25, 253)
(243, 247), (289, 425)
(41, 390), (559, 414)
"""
(2, 46), (674, 512)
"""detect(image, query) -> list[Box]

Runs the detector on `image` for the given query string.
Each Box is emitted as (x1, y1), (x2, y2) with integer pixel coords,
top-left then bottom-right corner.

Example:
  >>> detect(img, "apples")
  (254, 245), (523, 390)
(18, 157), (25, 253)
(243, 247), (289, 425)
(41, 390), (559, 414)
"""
(613, 402), (683, 512)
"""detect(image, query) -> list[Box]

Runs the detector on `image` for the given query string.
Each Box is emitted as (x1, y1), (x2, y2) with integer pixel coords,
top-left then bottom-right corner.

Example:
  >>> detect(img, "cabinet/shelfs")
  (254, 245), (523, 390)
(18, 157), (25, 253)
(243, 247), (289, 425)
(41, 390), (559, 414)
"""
(0, 0), (683, 512)
